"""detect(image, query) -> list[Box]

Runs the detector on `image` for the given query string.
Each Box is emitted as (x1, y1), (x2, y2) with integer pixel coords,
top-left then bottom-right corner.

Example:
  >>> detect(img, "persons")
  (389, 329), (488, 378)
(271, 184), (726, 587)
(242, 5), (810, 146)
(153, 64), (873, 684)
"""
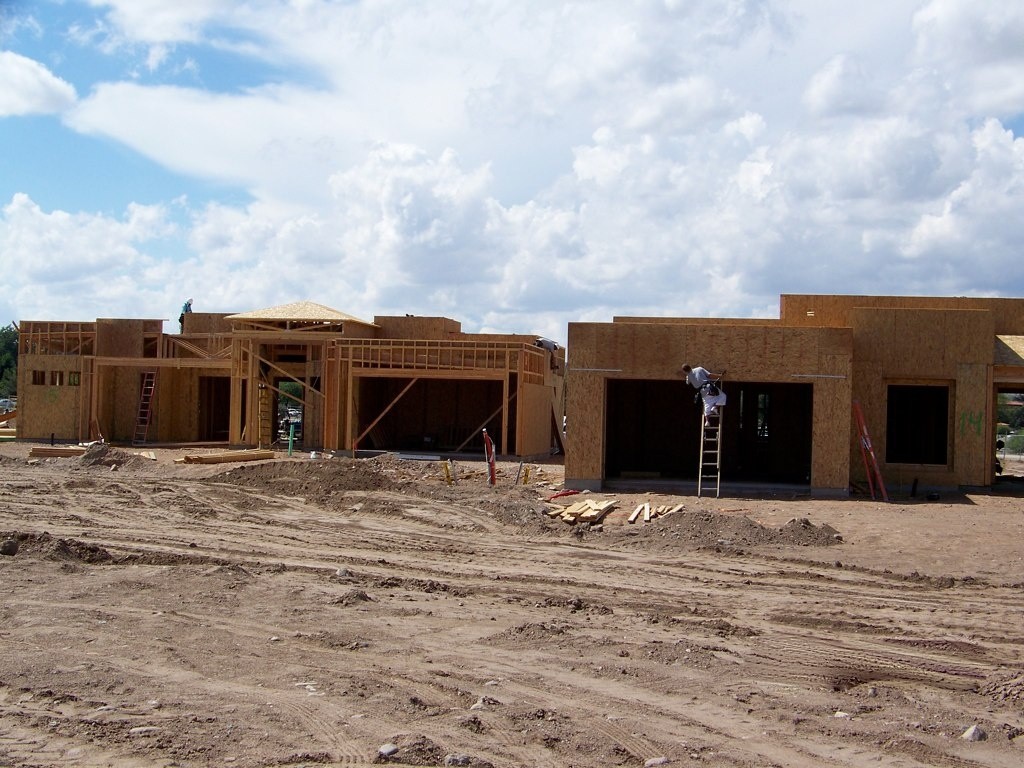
(533, 337), (559, 368)
(179, 298), (193, 334)
(681, 363), (726, 426)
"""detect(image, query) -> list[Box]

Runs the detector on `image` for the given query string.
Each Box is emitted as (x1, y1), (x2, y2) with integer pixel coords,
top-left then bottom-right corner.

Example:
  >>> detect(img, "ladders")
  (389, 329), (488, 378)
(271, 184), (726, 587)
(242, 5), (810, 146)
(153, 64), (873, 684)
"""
(130, 366), (159, 446)
(697, 379), (724, 500)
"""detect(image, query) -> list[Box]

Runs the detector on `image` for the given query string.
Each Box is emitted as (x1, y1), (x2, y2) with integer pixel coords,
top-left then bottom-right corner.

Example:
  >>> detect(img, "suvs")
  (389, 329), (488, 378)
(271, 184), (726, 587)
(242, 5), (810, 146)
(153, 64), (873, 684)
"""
(288, 409), (302, 417)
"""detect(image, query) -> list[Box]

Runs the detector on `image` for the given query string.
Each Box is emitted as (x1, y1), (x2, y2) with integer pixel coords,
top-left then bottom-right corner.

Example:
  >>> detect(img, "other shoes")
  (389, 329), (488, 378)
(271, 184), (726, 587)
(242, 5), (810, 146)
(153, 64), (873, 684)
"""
(705, 422), (710, 427)
(711, 407), (719, 415)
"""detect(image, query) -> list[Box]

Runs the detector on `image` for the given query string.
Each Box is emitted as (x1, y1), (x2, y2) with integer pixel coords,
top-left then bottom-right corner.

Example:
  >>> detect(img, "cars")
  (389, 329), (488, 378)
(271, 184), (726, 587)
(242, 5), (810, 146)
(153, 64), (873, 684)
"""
(0, 399), (16, 407)
